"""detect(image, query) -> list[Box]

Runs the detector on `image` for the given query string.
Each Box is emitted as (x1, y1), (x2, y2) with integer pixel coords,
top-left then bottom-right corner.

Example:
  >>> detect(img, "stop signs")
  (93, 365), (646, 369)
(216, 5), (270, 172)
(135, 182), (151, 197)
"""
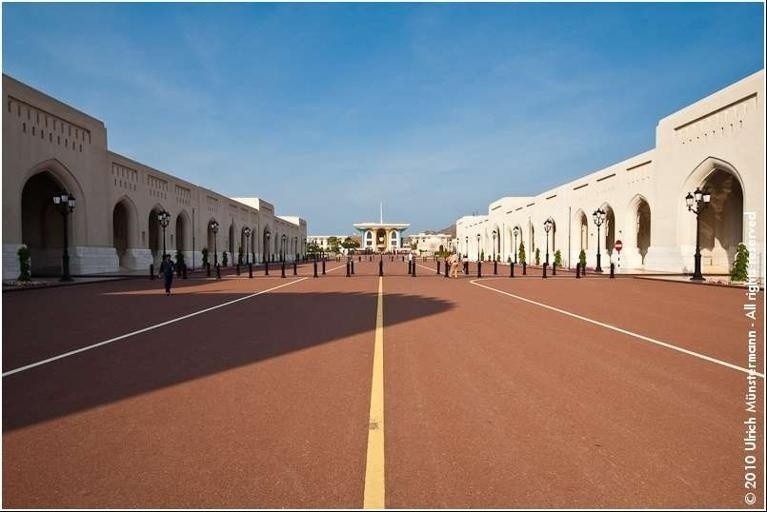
(614, 240), (622, 251)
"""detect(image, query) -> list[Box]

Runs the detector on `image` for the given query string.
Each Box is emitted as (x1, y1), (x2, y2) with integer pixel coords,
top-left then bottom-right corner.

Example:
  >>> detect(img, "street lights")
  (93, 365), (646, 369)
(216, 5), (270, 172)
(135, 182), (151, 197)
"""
(543, 218), (554, 266)
(446, 225), (520, 264)
(592, 208), (606, 273)
(210, 220), (307, 268)
(52, 187), (76, 283)
(685, 186), (712, 281)
(157, 210), (171, 273)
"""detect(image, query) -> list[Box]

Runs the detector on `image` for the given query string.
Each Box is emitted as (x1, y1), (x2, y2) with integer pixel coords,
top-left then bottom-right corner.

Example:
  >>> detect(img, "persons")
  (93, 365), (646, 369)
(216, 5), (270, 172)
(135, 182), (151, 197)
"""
(447, 251), (458, 278)
(175, 249), (186, 279)
(460, 256), (468, 272)
(157, 254), (174, 296)
